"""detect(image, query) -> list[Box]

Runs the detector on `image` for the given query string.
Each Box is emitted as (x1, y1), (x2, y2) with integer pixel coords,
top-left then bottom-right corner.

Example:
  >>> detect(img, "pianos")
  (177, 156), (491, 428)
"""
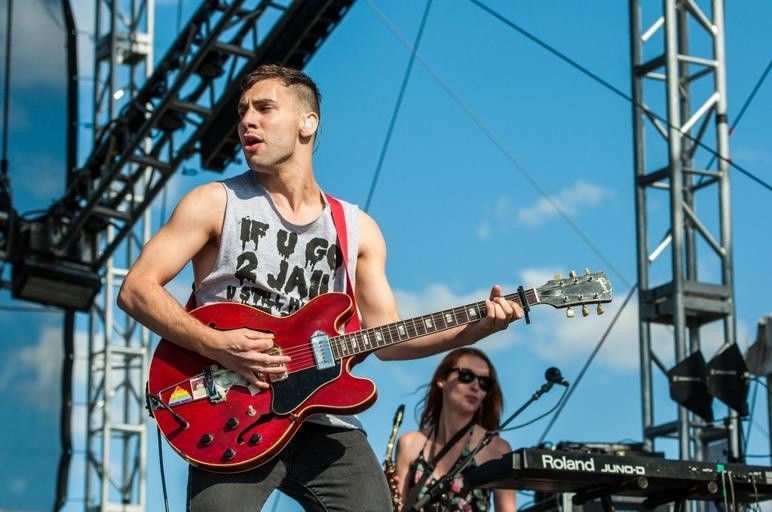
(464, 446), (772, 512)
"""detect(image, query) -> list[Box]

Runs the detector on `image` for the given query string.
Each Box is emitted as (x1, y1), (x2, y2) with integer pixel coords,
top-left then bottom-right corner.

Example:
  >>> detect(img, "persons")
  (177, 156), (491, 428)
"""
(116, 66), (525, 511)
(395, 347), (516, 511)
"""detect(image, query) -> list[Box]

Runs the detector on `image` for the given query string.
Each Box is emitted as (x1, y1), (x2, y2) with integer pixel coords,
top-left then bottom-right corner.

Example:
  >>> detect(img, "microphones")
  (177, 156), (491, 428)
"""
(545, 368), (571, 387)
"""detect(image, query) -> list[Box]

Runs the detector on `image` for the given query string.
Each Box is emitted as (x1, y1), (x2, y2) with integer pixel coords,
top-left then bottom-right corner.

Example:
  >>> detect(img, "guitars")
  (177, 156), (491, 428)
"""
(145, 268), (614, 475)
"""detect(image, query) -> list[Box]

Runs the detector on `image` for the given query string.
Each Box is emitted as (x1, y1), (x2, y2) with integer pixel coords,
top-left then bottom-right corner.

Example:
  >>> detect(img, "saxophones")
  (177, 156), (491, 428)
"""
(380, 404), (408, 511)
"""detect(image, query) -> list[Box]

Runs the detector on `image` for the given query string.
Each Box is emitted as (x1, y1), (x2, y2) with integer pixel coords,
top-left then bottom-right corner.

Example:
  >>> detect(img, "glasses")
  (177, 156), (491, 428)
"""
(448, 368), (496, 393)
